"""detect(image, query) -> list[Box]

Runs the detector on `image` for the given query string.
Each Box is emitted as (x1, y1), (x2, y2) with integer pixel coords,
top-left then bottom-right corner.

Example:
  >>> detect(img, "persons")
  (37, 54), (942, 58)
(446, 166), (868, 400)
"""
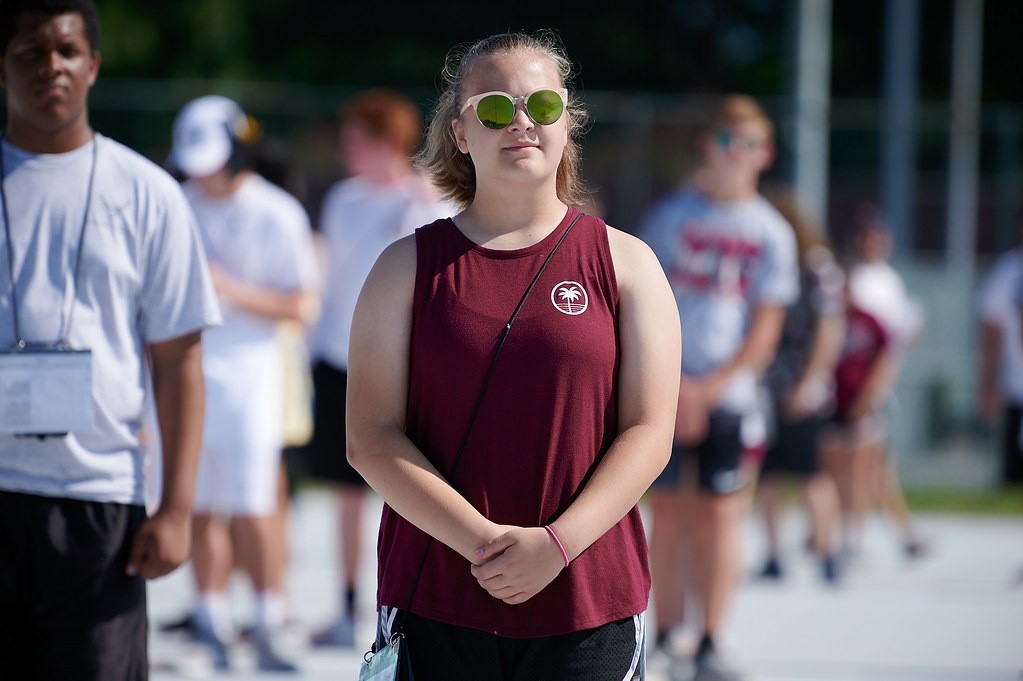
(145, 90), (930, 675)
(975, 241), (1023, 496)
(344, 34), (682, 681)
(0, 0), (228, 681)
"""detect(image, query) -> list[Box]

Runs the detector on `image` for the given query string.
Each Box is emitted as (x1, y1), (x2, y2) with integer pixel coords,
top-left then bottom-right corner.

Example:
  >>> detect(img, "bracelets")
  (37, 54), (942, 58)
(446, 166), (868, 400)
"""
(544, 525), (569, 567)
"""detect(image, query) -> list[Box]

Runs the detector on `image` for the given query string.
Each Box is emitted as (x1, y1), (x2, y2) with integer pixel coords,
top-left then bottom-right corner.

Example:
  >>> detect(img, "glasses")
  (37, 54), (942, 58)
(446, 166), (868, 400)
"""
(459, 87), (569, 130)
